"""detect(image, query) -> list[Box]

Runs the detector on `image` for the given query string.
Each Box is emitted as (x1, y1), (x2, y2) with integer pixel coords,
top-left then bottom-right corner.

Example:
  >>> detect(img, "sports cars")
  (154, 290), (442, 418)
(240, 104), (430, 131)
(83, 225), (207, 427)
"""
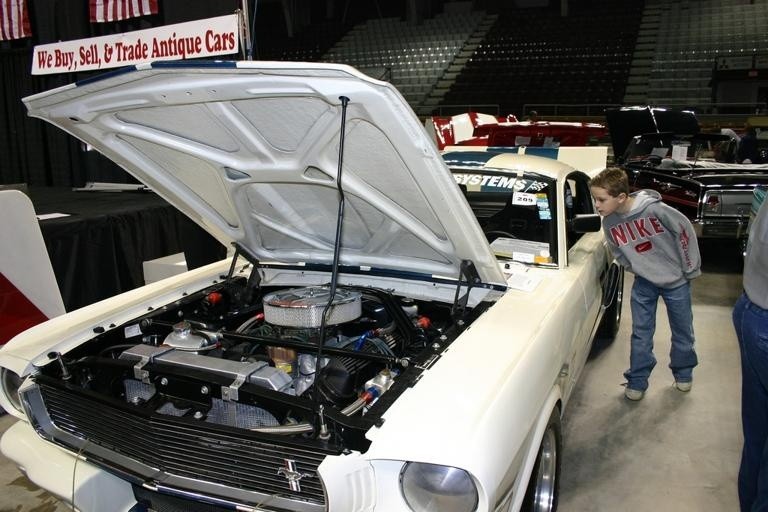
(0, 58), (626, 510)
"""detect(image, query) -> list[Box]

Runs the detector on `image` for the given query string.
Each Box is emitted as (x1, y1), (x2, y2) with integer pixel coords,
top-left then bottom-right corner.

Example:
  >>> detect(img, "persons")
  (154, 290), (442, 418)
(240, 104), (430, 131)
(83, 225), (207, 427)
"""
(709, 122), (741, 163)
(731, 192), (768, 512)
(586, 165), (702, 402)
(736, 126), (763, 164)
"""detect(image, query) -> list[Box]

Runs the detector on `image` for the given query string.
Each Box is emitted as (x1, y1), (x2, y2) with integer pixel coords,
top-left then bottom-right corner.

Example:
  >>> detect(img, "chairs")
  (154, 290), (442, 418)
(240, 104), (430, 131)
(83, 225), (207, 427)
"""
(483, 192), (551, 246)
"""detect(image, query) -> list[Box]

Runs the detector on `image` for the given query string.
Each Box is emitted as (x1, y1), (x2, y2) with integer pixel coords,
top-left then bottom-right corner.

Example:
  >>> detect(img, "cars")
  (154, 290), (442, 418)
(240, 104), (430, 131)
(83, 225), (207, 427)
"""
(603, 103), (768, 241)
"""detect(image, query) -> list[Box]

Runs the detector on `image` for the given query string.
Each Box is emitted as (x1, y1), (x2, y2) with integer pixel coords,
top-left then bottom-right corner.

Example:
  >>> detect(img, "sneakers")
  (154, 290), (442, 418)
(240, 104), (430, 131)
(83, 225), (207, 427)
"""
(676, 380), (692, 392)
(625, 380), (643, 401)
